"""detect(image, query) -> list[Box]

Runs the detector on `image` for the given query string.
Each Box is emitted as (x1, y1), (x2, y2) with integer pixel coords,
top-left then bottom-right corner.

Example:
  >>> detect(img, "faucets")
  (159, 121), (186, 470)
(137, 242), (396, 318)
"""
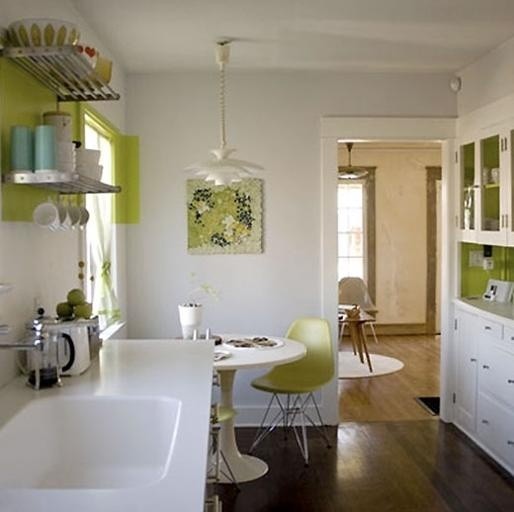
(0, 337), (46, 394)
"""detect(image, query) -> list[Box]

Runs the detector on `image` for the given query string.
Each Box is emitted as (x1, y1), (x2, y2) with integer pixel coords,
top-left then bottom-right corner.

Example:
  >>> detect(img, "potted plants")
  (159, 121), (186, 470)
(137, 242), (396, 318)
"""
(177, 269), (219, 340)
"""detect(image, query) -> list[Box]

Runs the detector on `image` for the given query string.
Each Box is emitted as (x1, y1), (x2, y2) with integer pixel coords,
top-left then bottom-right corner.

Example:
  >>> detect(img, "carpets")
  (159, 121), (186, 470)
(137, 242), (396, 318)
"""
(337, 350), (405, 379)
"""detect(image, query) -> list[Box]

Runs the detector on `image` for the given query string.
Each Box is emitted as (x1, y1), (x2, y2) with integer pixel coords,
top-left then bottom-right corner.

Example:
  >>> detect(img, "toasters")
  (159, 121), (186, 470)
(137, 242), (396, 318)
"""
(23, 317), (102, 377)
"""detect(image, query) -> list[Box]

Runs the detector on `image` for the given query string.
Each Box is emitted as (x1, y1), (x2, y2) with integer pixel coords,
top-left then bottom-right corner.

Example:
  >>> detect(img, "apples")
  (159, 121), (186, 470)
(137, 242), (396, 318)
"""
(56, 287), (92, 321)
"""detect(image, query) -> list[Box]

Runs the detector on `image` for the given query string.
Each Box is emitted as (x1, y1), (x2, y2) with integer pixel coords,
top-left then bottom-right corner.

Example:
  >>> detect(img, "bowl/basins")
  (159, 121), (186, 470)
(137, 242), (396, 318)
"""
(56, 140), (103, 185)
(343, 308), (360, 319)
(7, 17), (113, 92)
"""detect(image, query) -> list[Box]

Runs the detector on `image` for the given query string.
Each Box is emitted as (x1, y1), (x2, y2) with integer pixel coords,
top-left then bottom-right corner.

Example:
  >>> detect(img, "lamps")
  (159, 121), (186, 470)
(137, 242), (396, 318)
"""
(338, 139), (371, 182)
(180, 39), (268, 191)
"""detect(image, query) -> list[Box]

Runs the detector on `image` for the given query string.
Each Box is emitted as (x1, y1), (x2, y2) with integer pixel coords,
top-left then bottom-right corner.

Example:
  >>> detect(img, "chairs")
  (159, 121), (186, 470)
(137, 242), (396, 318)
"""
(243, 316), (335, 468)
(335, 275), (381, 349)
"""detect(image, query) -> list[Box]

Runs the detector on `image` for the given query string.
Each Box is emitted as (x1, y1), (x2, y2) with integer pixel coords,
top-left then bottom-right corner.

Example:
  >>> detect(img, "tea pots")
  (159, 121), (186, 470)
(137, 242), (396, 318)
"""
(17, 307), (77, 390)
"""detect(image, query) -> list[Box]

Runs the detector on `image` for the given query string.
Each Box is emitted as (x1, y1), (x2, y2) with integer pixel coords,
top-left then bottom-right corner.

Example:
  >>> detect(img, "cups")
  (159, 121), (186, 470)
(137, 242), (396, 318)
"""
(33, 195), (92, 232)
(9, 108), (74, 175)
(481, 164), (500, 185)
(176, 302), (204, 339)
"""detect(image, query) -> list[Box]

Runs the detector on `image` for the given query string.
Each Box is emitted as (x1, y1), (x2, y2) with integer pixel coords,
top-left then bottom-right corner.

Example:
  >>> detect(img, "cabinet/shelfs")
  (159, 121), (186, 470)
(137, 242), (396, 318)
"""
(0, 43), (122, 197)
(447, 121), (514, 482)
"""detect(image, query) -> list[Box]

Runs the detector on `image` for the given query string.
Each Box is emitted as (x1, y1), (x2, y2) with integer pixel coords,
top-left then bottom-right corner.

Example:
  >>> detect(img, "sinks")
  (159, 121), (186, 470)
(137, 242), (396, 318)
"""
(0, 384), (196, 511)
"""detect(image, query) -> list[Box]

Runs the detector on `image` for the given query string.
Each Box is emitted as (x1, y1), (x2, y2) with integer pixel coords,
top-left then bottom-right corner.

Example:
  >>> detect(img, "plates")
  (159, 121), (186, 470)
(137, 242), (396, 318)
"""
(223, 334), (285, 352)
